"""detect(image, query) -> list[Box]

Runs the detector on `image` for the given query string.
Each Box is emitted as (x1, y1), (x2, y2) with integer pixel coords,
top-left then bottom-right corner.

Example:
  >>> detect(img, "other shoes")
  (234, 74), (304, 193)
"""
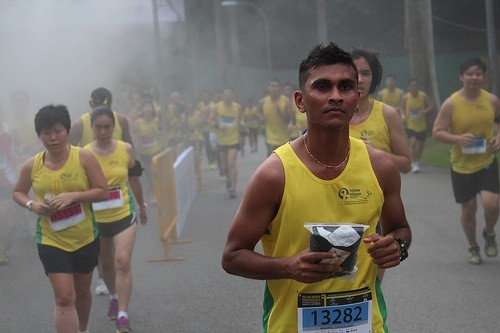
(411, 162), (420, 171)
(96, 278), (109, 294)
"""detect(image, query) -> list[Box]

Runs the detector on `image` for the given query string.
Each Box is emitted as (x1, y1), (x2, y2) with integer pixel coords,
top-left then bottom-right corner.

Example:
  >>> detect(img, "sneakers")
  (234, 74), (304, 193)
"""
(108, 299), (117, 319)
(483, 229), (498, 257)
(116, 317), (131, 333)
(468, 244), (481, 264)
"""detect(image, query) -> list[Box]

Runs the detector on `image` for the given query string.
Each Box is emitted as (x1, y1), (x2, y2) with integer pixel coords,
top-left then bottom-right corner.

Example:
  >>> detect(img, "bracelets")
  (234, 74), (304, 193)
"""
(139, 203), (147, 211)
(394, 238), (408, 261)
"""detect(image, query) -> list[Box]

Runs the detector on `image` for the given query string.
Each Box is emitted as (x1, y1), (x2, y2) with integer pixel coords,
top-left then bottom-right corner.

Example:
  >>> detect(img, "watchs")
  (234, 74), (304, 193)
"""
(25, 200), (33, 211)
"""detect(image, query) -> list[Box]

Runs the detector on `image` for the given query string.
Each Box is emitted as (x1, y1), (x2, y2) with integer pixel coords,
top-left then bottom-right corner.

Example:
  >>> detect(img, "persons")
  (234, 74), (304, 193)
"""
(401, 78), (435, 173)
(433, 57), (500, 265)
(351, 49), (412, 280)
(70, 88), (135, 295)
(83, 107), (148, 333)
(137, 87), (257, 199)
(257, 79), (293, 158)
(221, 41), (412, 333)
(378, 75), (404, 107)
(13, 105), (109, 333)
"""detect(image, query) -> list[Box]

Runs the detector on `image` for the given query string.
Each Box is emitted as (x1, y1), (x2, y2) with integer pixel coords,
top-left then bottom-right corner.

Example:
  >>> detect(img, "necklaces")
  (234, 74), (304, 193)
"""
(303, 133), (351, 171)
